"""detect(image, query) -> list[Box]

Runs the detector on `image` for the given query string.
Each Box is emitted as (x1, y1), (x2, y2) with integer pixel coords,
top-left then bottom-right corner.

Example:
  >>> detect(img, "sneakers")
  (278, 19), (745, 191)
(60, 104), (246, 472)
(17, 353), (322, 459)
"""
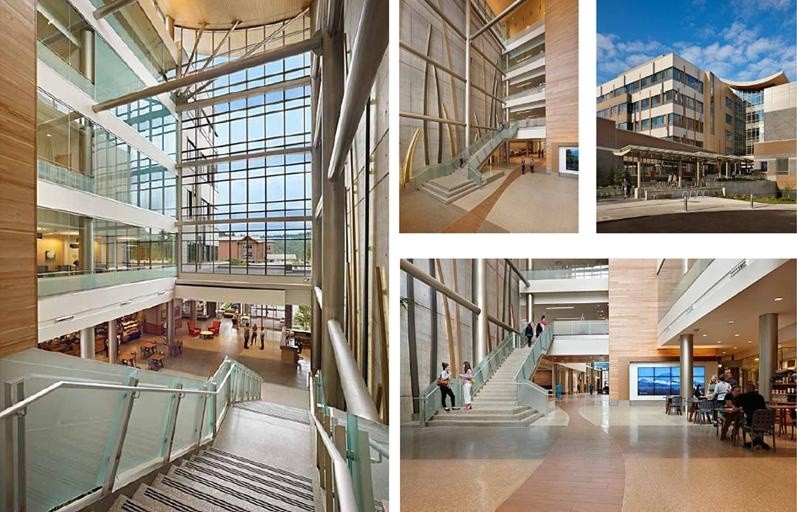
(444, 407), (450, 411)
(718, 430), (739, 440)
(742, 438), (769, 450)
(465, 405), (472, 410)
(452, 407), (460, 410)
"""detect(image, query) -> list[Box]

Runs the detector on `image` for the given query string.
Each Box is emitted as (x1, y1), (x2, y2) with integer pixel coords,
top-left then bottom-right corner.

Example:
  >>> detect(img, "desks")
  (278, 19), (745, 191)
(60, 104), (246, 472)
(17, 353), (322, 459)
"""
(196, 330), (214, 339)
(117, 337), (164, 372)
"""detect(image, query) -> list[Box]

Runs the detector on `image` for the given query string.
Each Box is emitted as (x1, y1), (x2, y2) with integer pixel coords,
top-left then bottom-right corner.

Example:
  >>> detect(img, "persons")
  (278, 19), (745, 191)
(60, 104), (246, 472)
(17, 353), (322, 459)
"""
(440, 363), (460, 410)
(460, 361), (473, 410)
(672, 172), (677, 183)
(622, 178), (628, 199)
(668, 173), (672, 182)
(251, 324), (257, 345)
(520, 154), (526, 175)
(529, 159), (535, 174)
(694, 373), (770, 451)
(499, 121), (504, 131)
(460, 148), (464, 167)
(260, 327), (265, 349)
(524, 315), (548, 347)
(243, 322), (250, 349)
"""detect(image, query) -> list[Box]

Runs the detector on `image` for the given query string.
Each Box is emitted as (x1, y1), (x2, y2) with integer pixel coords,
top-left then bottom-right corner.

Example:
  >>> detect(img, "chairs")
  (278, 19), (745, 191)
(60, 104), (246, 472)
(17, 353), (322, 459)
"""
(186, 318), (202, 337)
(207, 319), (222, 337)
(663, 390), (796, 454)
(296, 341), (304, 360)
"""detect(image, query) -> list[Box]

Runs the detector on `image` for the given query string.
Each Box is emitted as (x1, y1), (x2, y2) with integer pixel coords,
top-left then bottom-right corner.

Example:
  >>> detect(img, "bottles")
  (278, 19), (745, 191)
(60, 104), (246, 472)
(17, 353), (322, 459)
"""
(771, 372), (796, 403)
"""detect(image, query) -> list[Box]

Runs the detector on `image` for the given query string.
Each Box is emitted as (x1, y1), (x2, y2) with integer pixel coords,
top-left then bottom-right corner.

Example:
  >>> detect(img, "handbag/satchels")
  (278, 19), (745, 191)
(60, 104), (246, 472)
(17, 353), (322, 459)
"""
(437, 377), (448, 386)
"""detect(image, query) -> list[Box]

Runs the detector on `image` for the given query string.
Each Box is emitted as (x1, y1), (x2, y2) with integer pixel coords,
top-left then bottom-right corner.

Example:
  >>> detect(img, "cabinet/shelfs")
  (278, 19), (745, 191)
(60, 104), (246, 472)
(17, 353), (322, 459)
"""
(768, 380), (796, 397)
